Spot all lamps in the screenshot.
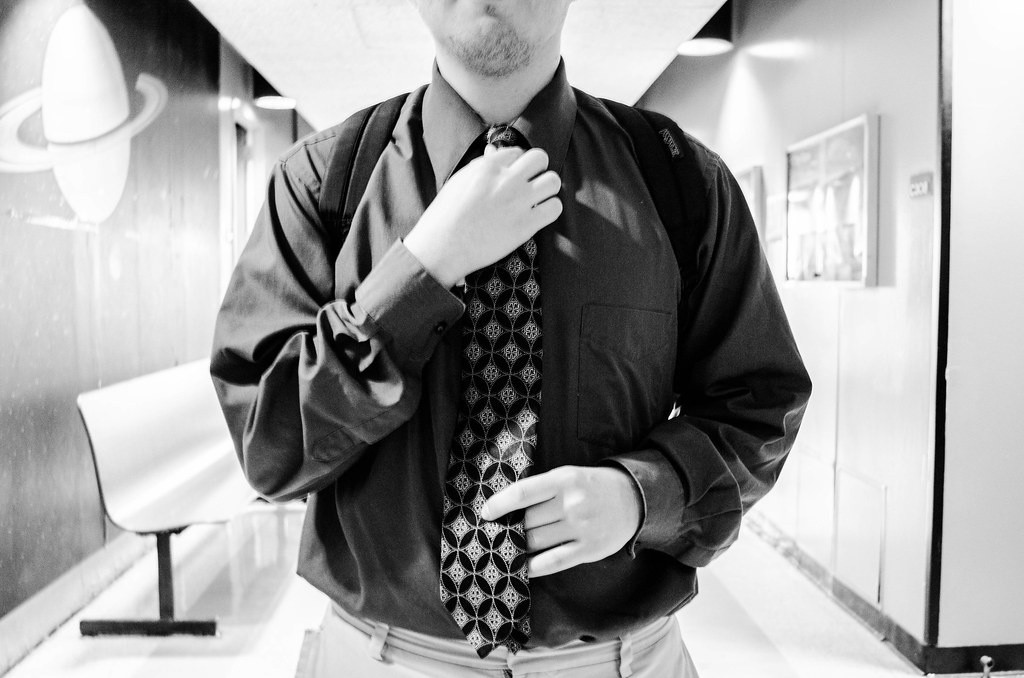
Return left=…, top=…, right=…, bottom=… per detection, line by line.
left=252, top=68, right=296, bottom=110
left=676, top=0, right=733, bottom=57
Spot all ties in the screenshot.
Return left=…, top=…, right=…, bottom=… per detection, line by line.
left=439, top=123, right=545, bottom=659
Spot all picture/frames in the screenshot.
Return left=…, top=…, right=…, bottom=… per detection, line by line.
left=734, top=165, right=762, bottom=237
left=783, top=112, right=878, bottom=291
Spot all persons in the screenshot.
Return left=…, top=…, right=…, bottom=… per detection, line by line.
left=209, top=0, right=812, bottom=677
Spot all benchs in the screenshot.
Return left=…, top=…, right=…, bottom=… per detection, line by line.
left=77, top=357, right=302, bottom=637
